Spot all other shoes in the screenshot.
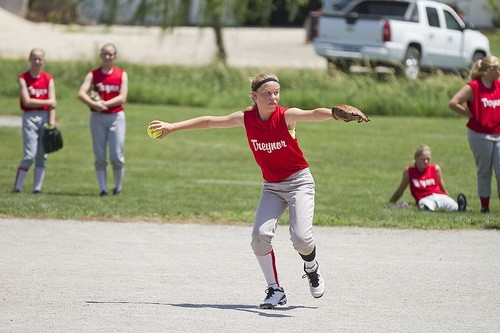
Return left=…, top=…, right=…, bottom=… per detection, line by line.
left=480, top=207, right=491, bottom=212
left=113, top=188, right=123, bottom=195
left=100, top=190, right=108, bottom=196
left=457, top=193, right=467, bottom=212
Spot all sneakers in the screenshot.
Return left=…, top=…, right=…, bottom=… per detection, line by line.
left=302, top=260, right=325, bottom=298
left=260, top=287, right=288, bottom=309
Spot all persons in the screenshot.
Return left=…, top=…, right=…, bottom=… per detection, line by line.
left=78, top=43, right=128, bottom=196
left=449, top=56, right=500, bottom=212
left=12, top=47, right=57, bottom=192
left=389, top=143, right=467, bottom=212
left=148, top=72, right=370, bottom=309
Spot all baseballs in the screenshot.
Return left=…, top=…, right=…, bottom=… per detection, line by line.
left=146, top=123, right=162, bottom=139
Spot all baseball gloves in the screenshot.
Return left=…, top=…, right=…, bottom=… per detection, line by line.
left=41, top=124, right=63, bottom=154
left=332, top=104, right=371, bottom=123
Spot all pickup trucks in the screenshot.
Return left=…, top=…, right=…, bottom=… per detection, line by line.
left=306, top=0, right=490, bottom=82
